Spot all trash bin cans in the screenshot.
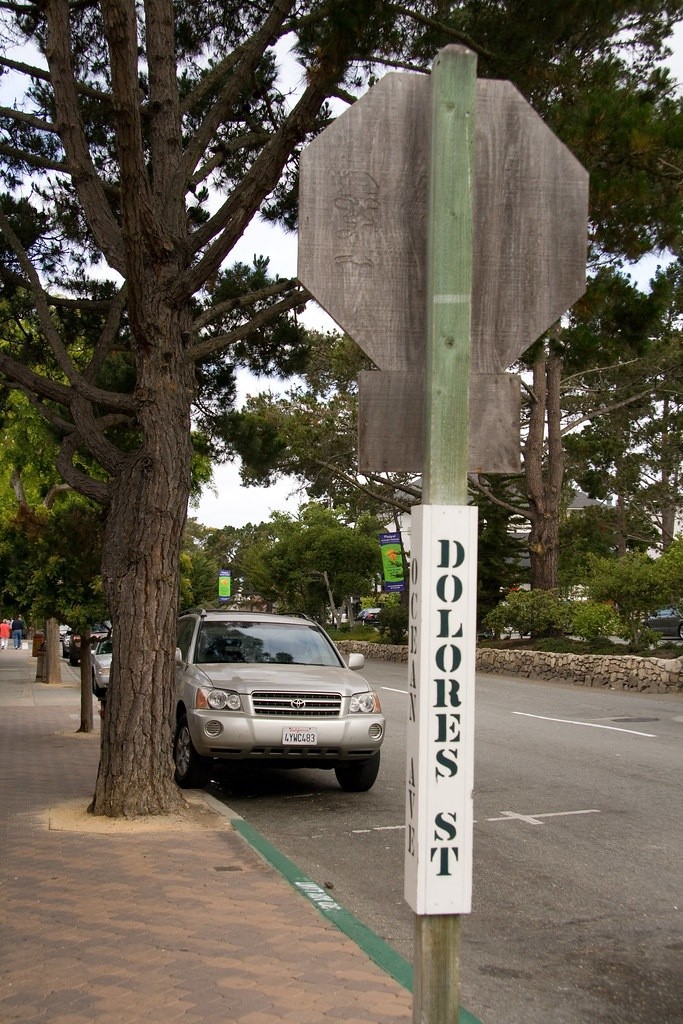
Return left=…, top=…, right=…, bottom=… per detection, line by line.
left=32, top=627, right=44, bottom=657
left=35, top=641, right=47, bottom=682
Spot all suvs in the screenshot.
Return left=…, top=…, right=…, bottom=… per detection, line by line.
left=176, top=608, right=383, bottom=794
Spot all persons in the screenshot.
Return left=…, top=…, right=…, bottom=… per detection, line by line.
left=10, top=616, right=23, bottom=649
left=0, top=619, right=10, bottom=649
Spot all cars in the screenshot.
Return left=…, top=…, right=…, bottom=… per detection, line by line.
left=639, top=608, right=683, bottom=639
left=353, top=608, right=383, bottom=628
left=60, top=624, right=83, bottom=666
left=90, top=635, right=113, bottom=696
